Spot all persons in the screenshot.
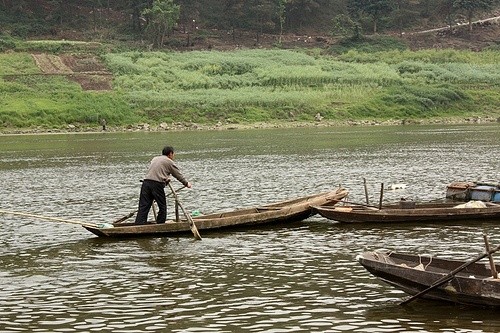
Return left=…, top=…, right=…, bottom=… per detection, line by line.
left=101, top=119, right=107, bottom=130
left=135, top=147, right=191, bottom=224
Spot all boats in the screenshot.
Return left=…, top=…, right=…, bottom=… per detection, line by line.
left=355, top=249, right=500, bottom=309
left=309, top=180, right=500, bottom=222
left=82, top=186, right=349, bottom=236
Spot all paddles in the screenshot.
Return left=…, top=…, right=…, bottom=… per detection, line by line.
left=114, top=186, right=186, bottom=223
left=398, top=245, right=500, bottom=306
left=168, top=182, right=201, bottom=241
left=483, top=234, right=498, bottom=278
